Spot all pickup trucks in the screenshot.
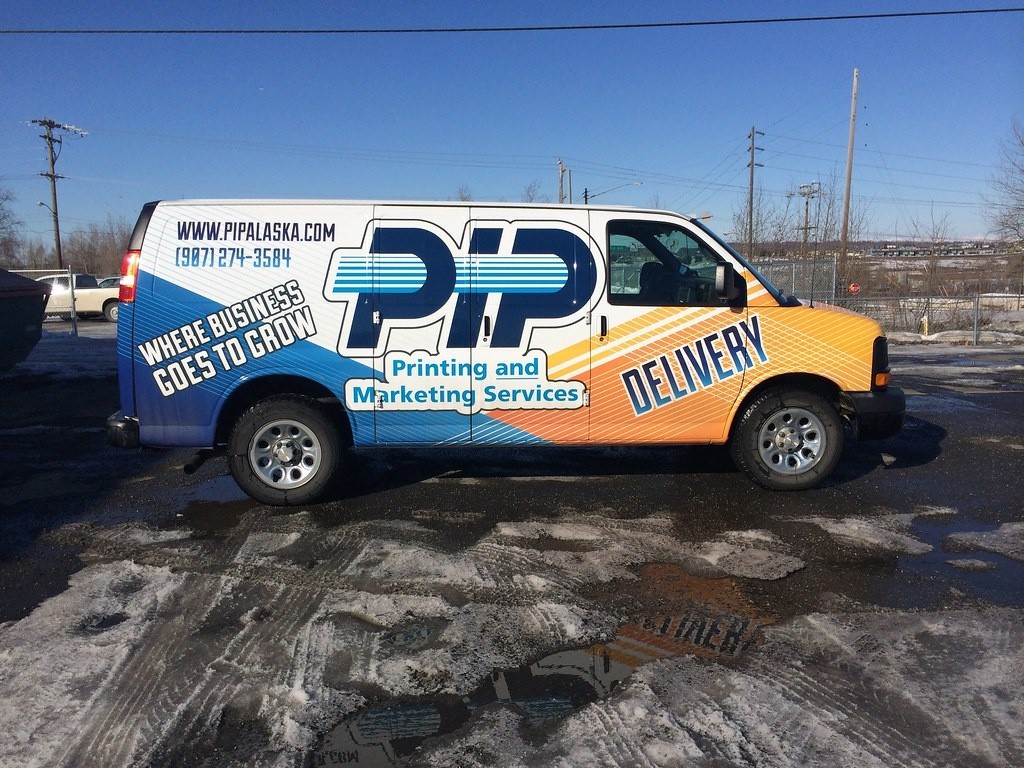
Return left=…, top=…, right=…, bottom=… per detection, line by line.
left=33, top=274, right=120, bottom=323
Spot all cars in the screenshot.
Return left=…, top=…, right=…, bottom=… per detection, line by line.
left=98, top=276, right=120, bottom=288
left=0, top=267, right=53, bottom=371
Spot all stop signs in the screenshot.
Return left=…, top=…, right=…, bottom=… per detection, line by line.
left=849, top=283, right=861, bottom=295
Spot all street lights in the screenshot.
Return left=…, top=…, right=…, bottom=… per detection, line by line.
left=798, top=179, right=821, bottom=261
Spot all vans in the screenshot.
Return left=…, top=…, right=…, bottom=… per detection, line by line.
left=102, top=197, right=892, bottom=506
